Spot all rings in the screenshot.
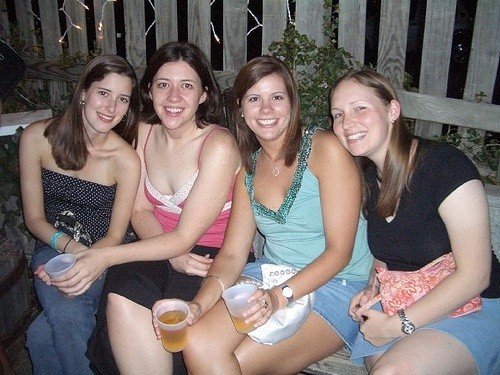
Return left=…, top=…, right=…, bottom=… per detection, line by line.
left=262, top=299, right=268, bottom=309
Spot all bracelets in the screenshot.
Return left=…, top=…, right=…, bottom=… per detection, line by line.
left=62, top=236, right=74, bottom=254
left=50, top=230, right=65, bottom=250
left=201, top=275, right=225, bottom=299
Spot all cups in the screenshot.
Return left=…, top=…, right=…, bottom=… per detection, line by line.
left=44, top=253, right=79, bottom=298
left=222, top=284, right=258, bottom=333
left=154, top=301, right=190, bottom=353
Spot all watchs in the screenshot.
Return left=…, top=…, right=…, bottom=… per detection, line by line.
left=279, top=282, right=295, bottom=306
left=397, top=308, right=415, bottom=335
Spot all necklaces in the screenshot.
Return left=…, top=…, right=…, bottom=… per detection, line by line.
left=261, top=147, right=286, bottom=178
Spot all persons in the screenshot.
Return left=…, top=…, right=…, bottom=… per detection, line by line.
left=104, top=41, right=255, bottom=375
left=326, top=68, right=500, bottom=375
left=151, top=55, right=377, bottom=375
left=16, top=53, right=142, bottom=375
